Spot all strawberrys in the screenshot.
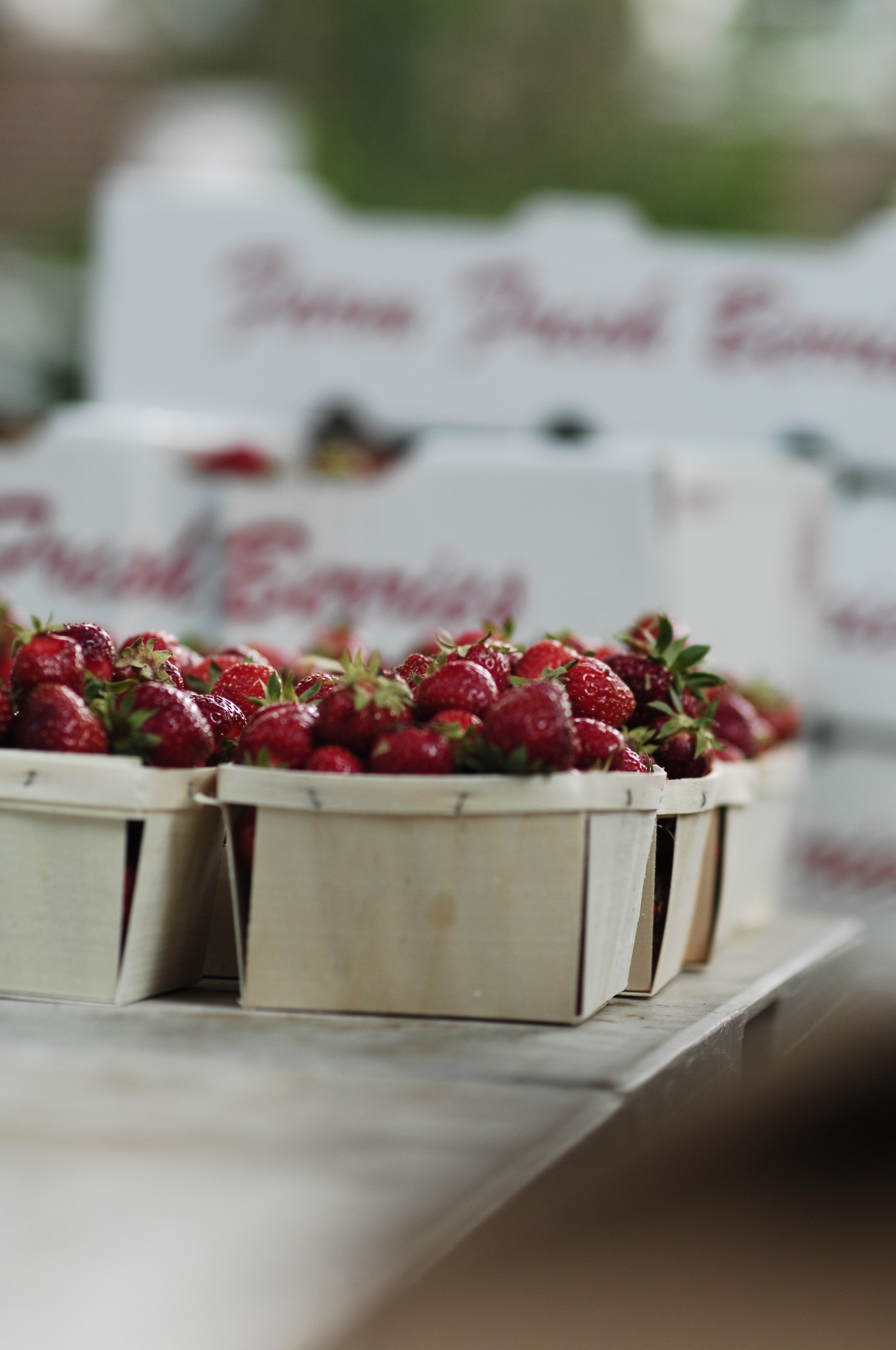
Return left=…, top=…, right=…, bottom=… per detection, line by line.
left=0, top=608, right=802, bottom=774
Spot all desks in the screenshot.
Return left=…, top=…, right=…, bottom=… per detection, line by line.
left=0, top=914, right=877, bottom=1350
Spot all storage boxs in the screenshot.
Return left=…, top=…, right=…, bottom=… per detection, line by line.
left=0, top=735, right=809, bottom=1034
